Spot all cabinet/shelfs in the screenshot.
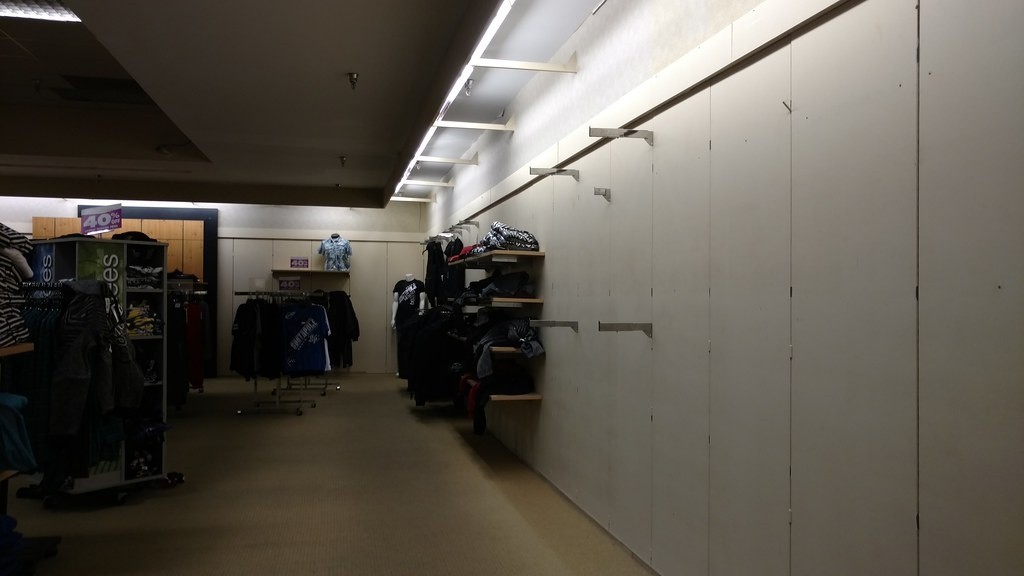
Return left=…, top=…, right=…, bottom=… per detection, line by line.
left=0, top=343, right=36, bottom=516
left=23, top=238, right=170, bottom=494
left=447, top=247, right=546, bottom=401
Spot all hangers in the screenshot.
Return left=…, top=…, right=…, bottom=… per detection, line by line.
left=22, top=281, right=66, bottom=311
left=247, top=291, right=312, bottom=304
